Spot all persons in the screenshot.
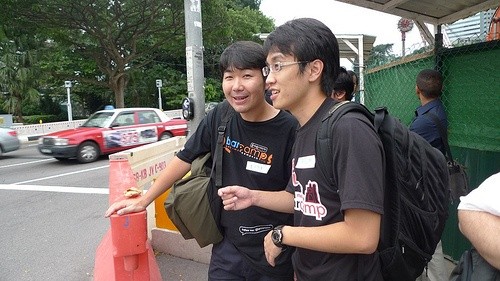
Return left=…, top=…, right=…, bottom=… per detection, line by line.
left=104, top=40, right=299, bottom=281
left=406, top=69, right=448, bottom=159
left=458, top=170, right=500, bottom=271
left=218, top=18, right=389, bottom=281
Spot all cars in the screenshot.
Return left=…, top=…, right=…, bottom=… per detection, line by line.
left=0, top=128, right=22, bottom=159
left=37, top=107, right=187, bottom=164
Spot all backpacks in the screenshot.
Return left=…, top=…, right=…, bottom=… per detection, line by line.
left=318, top=100, right=451, bottom=281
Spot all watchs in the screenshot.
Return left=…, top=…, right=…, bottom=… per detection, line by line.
left=271, top=224, right=285, bottom=247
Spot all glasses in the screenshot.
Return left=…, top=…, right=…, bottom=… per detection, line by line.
left=262, top=61, right=312, bottom=78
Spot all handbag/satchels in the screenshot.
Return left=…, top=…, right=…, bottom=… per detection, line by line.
left=447, top=158, right=469, bottom=205
left=164, top=152, right=223, bottom=249
left=449, top=248, right=500, bottom=281
